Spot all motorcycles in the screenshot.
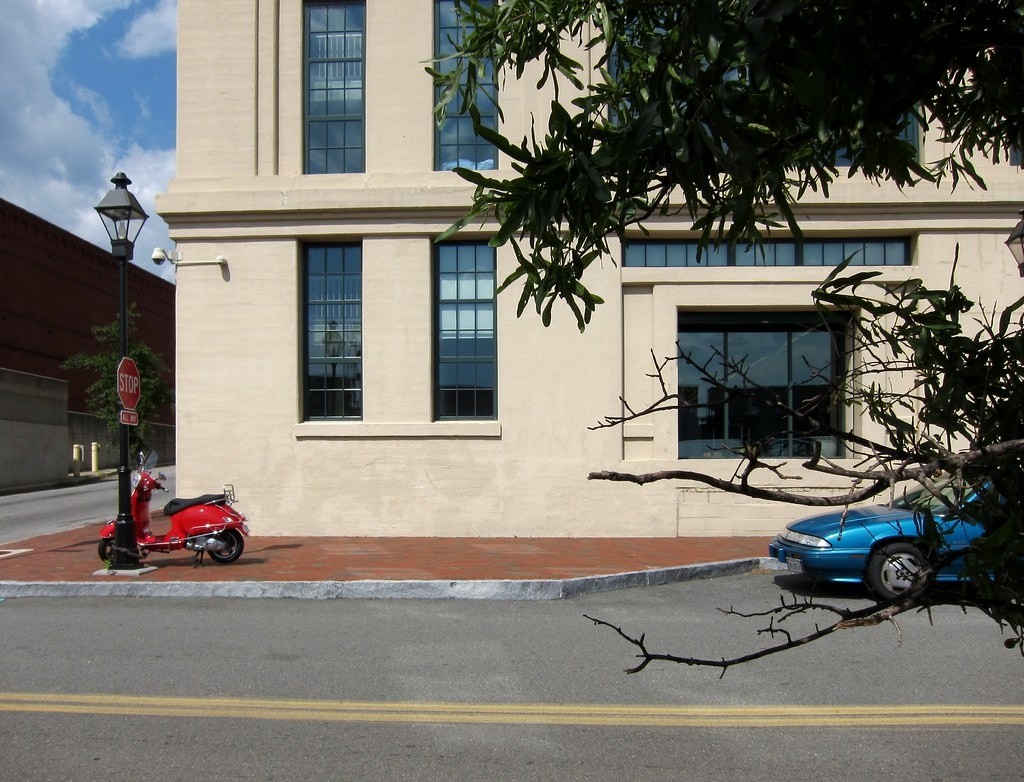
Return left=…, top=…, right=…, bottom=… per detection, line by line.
left=97, top=448, right=251, bottom=571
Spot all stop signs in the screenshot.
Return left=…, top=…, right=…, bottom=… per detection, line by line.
left=117, top=357, right=140, bottom=410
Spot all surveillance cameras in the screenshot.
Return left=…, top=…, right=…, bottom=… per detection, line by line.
left=151, top=251, right=166, bottom=266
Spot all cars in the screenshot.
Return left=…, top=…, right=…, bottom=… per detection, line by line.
left=766, top=471, right=1007, bottom=606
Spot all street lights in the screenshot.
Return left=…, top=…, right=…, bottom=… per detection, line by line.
left=94, top=171, right=152, bottom=572
